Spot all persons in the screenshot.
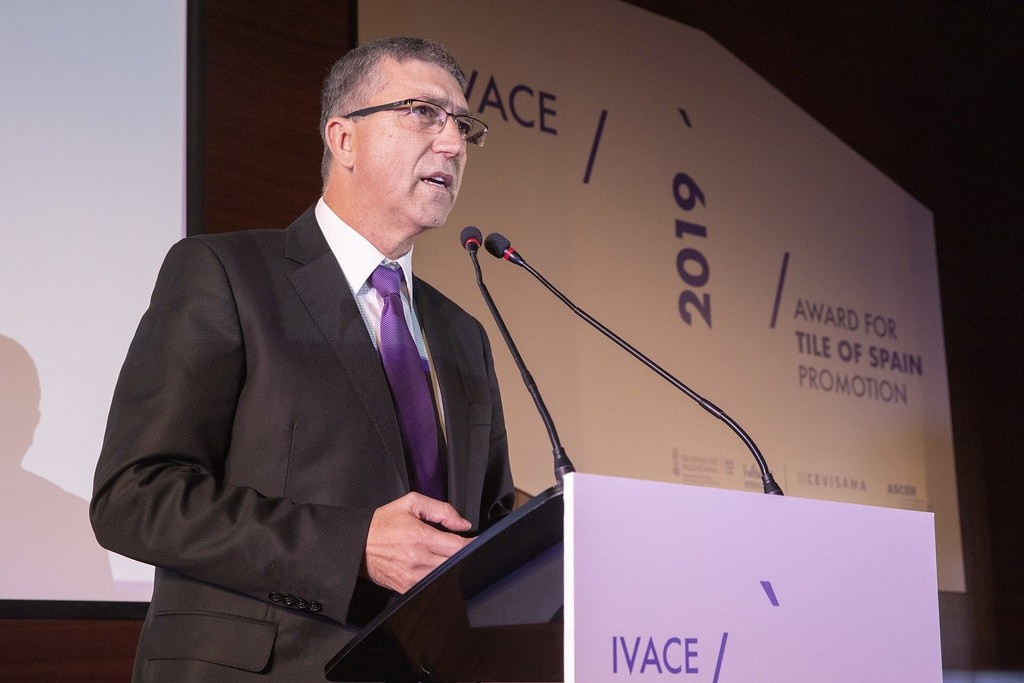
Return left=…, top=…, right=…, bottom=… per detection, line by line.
left=89, top=34, right=517, bottom=683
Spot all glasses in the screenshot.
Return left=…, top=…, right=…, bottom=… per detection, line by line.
left=340, top=99, right=488, bottom=148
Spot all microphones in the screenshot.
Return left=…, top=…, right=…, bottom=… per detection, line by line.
left=459, top=226, right=575, bottom=482
left=484, top=233, right=785, bottom=498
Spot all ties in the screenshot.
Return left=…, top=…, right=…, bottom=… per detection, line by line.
left=368, top=266, right=445, bottom=497
